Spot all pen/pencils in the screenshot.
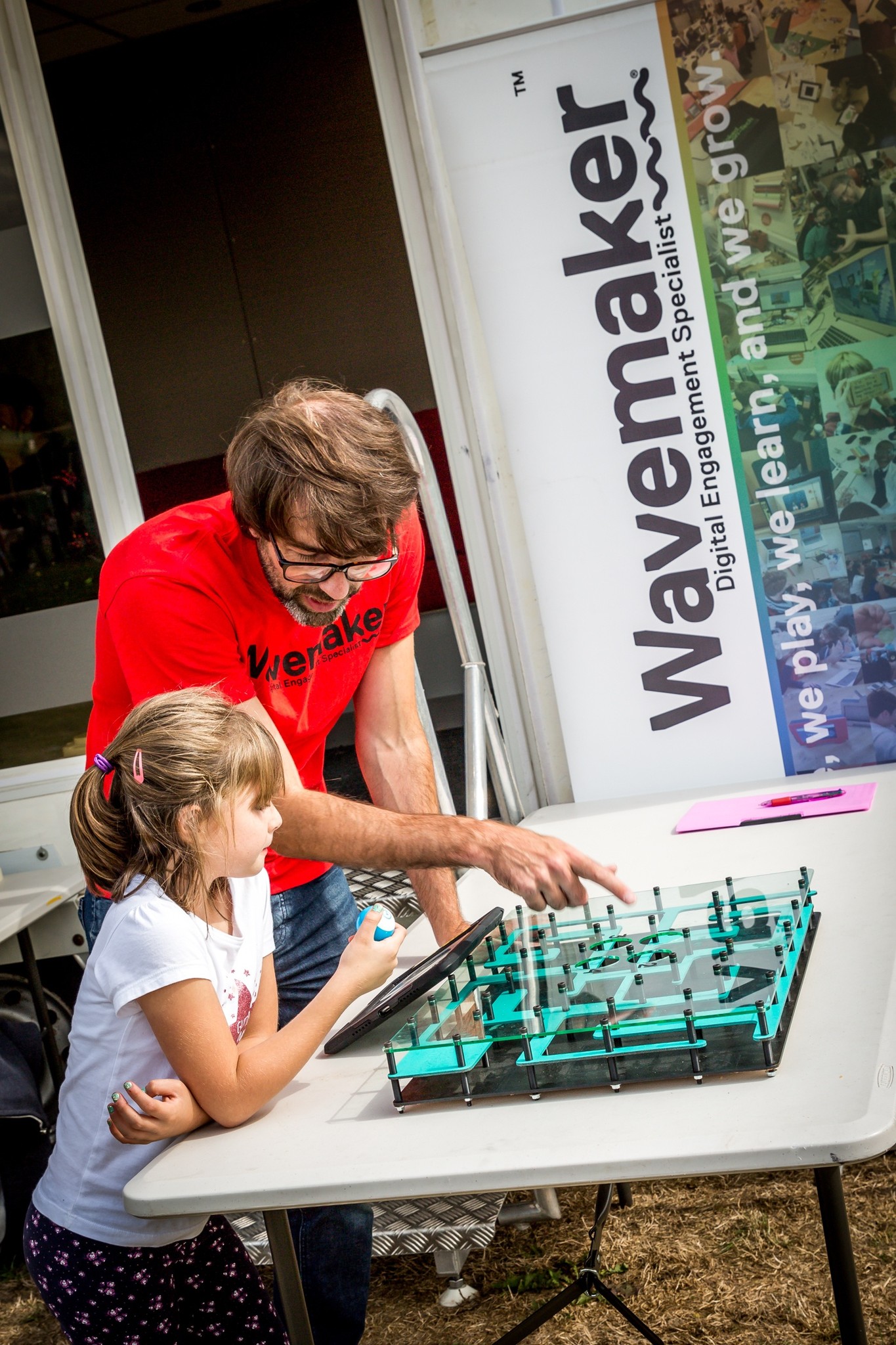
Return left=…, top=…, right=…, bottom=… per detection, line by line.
left=758, top=789, right=846, bottom=807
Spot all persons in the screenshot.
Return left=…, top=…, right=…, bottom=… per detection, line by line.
left=21, top=684, right=409, bottom=1345
left=676, top=0, right=896, bottom=772
left=76, top=377, right=639, bottom=1345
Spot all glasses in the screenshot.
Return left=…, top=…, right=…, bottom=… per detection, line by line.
left=268, top=523, right=398, bottom=582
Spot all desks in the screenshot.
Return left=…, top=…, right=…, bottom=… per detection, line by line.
left=0, top=858, right=88, bottom=1122
left=126, top=766, right=896, bottom=1345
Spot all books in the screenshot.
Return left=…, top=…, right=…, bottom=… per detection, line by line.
left=674, top=782, right=877, bottom=833
